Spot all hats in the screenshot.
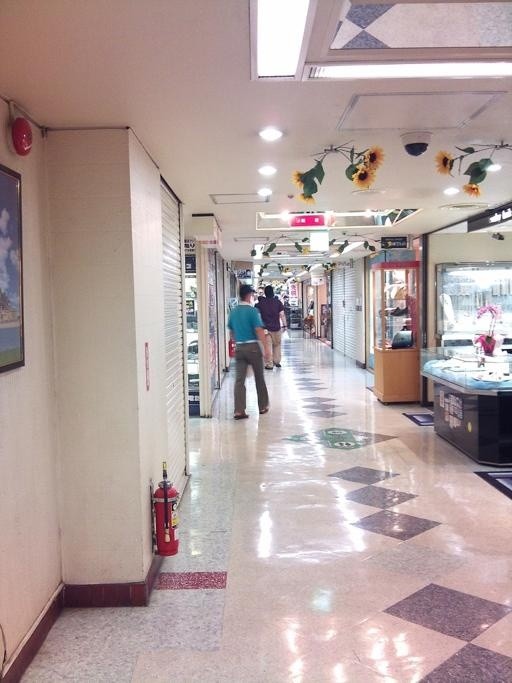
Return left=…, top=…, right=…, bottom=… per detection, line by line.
left=239, top=285, right=257, bottom=295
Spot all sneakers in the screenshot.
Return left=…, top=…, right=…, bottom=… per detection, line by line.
left=275, top=363, right=282, bottom=367
left=264, top=366, right=274, bottom=369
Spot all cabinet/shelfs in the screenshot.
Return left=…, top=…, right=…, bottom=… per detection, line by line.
left=372, top=262, right=420, bottom=403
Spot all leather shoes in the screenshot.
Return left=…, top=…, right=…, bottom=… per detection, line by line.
left=390, top=307, right=409, bottom=316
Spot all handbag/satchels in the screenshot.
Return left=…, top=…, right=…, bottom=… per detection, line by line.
left=392, top=325, right=413, bottom=349
left=390, top=286, right=408, bottom=300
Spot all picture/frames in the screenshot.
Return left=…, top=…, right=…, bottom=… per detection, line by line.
left=0, top=164, right=25, bottom=373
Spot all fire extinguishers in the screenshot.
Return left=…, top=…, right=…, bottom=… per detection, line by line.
left=229, top=337, right=236, bottom=357
left=151, top=462, right=179, bottom=557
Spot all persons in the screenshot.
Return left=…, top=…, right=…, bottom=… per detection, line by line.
left=255, top=286, right=288, bottom=370
left=227, top=284, right=269, bottom=420
left=255, top=295, right=291, bottom=332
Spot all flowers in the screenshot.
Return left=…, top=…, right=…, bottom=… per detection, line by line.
left=290, top=146, right=385, bottom=207
left=252, top=235, right=311, bottom=258
left=327, top=234, right=382, bottom=258
left=434, top=142, right=512, bottom=200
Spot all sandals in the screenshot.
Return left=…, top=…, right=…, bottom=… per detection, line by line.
left=259, top=406, right=270, bottom=414
left=234, top=413, right=249, bottom=420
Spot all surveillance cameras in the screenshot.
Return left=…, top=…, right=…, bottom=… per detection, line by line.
left=491, top=233, right=504, bottom=240
left=400, top=132, right=434, bottom=156
